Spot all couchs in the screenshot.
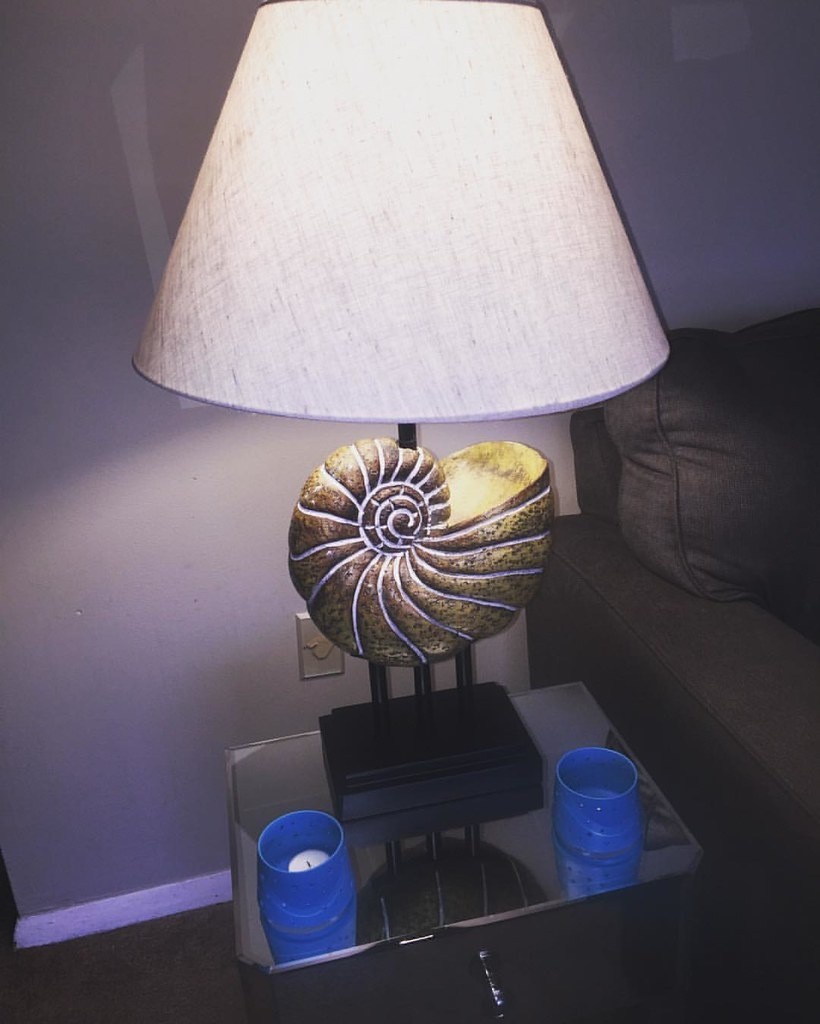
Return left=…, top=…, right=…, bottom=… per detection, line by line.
left=524, top=307, right=820, bottom=1024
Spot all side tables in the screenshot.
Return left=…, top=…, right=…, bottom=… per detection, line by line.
left=225, top=683, right=701, bottom=1024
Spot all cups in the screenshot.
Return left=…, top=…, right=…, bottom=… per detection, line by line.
left=257, top=810, right=357, bottom=930
left=550, top=746, right=643, bottom=857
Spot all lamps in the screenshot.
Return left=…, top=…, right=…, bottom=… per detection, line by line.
left=133, top=0, right=670, bottom=823
